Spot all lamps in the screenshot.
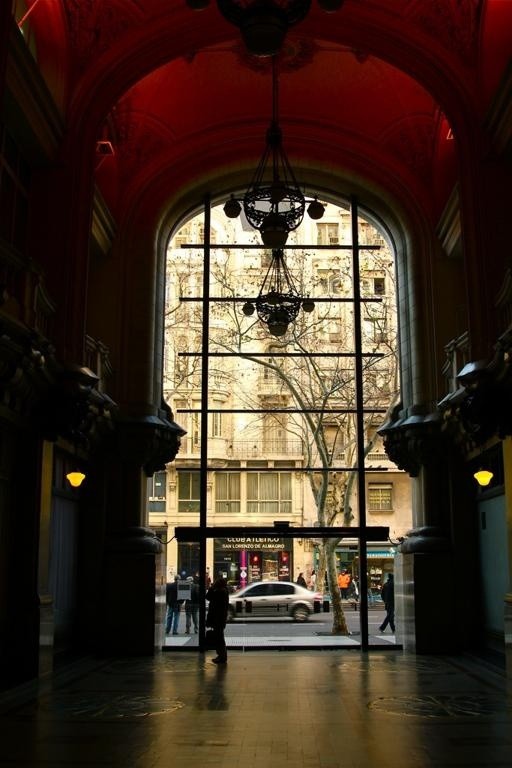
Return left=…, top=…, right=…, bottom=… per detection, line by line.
left=473, top=424, right=495, bottom=487
left=185, top=58, right=345, bottom=246
left=186, top=1, right=345, bottom=58
left=186, top=246, right=345, bottom=335
left=59, top=424, right=89, bottom=488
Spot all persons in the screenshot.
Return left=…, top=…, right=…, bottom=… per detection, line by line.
left=311, top=570, right=317, bottom=585
left=379, top=574, right=395, bottom=633
left=206, top=578, right=229, bottom=664
left=297, top=573, right=307, bottom=589
left=338, top=568, right=351, bottom=599
left=367, top=571, right=373, bottom=603
left=185, top=575, right=200, bottom=634
left=166, top=574, right=184, bottom=635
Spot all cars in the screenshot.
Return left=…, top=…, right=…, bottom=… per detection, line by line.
left=205, top=579, right=326, bottom=625
